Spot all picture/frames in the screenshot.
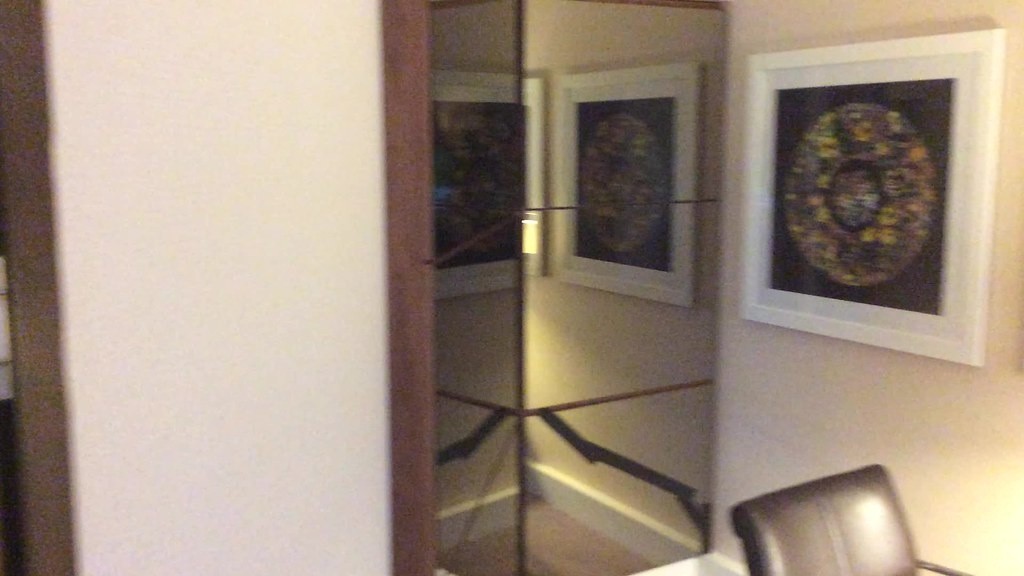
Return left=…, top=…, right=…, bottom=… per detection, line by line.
left=523, top=79, right=549, bottom=286
left=547, top=65, right=705, bottom=304
left=732, top=26, right=1010, bottom=368
left=430, top=71, right=523, bottom=297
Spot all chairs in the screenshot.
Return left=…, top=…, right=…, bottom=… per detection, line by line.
left=728, top=461, right=973, bottom=576
